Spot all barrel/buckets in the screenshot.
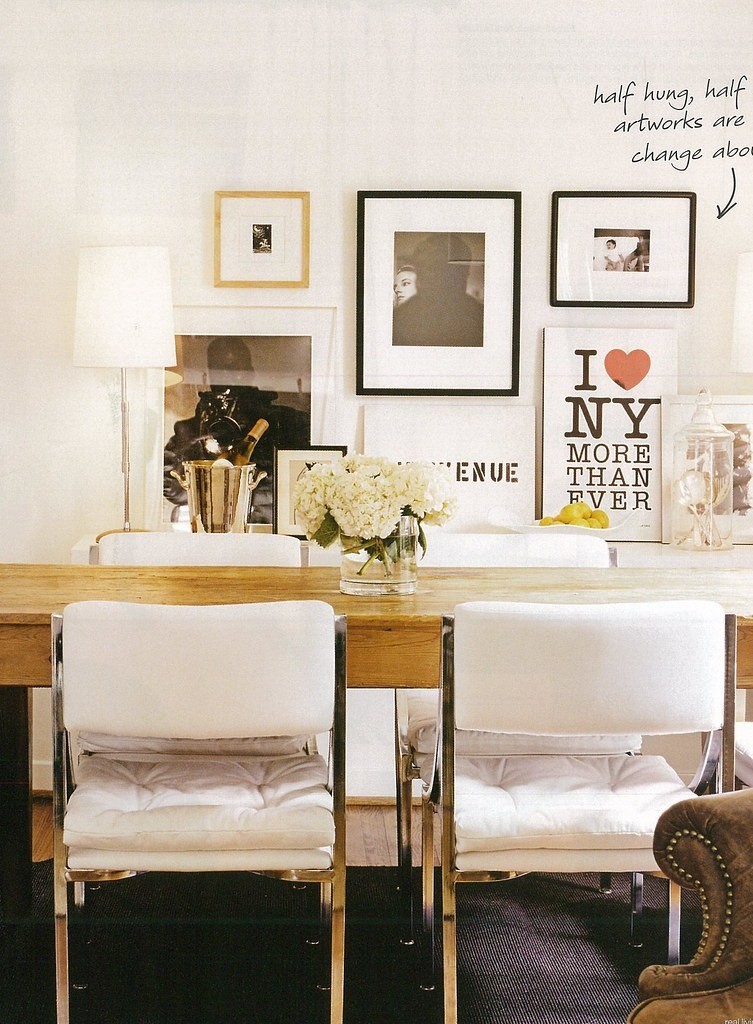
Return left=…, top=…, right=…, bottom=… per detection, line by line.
left=170, top=459, right=267, bottom=534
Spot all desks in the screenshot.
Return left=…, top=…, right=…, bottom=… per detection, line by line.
left=1, top=563, right=753, bottom=957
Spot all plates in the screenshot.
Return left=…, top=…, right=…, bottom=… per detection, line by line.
left=506, top=509, right=636, bottom=540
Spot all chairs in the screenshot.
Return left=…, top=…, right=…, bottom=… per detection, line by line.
left=71, top=531, right=321, bottom=945
left=421, top=601, right=735, bottom=1024
left=52, top=599, right=347, bottom=1024
left=392, top=530, right=650, bottom=950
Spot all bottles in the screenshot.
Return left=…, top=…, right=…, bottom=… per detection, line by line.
left=210, top=419, right=269, bottom=467
left=671, top=386, right=734, bottom=551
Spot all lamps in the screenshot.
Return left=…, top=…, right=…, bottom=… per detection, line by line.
left=71, top=245, right=179, bottom=542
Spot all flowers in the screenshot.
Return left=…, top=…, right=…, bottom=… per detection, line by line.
left=293, top=454, right=460, bottom=581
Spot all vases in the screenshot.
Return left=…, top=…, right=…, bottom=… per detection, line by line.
left=339, top=516, right=419, bottom=595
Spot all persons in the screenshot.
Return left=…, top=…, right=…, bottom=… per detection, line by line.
left=165, top=337, right=310, bottom=527
left=604, top=240, right=624, bottom=271
left=392, top=233, right=484, bottom=348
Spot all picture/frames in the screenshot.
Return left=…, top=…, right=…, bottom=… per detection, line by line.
left=549, top=191, right=696, bottom=308
left=214, top=190, right=310, bottom=287
left=358, top=190, right=522, bottom=398
